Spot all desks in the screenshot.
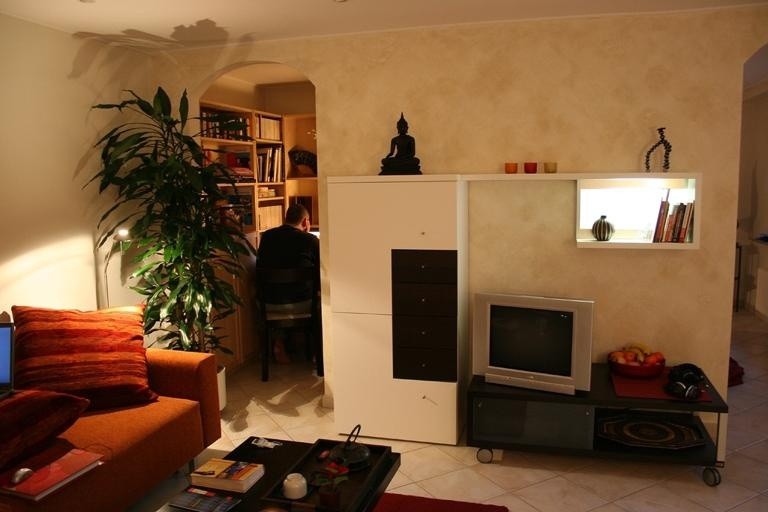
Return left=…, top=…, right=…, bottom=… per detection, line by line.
left=462, top=363, right=731, bottom=490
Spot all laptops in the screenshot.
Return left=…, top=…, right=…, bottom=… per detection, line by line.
left=0, top=322, right=15, bottom=399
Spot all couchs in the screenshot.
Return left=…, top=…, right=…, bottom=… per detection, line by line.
left=0, top=343, right=222, bottom=510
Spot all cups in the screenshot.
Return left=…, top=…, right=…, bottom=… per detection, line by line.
left=281, top=473, right=307, bottom=499
left=505, top=162, right=557, bottom=174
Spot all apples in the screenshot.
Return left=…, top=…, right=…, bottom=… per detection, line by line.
left=645, top=356, right=656, bottom=366
left=651, top=352, right=664, bottom=362
left=609, top=351, right=640, bottom=366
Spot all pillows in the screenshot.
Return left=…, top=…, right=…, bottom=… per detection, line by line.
left=0, top=302, right=159, bottom=473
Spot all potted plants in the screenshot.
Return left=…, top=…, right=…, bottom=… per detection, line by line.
left=78, top=84, right=259, bottom=422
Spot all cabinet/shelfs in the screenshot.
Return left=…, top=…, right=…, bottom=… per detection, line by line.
left=197, top=97, right=319, bottom=377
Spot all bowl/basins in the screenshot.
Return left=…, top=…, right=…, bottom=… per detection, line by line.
left=608, top=350, right=666, bottom=381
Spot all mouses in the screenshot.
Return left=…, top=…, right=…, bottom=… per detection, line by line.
left=11, top=468, right=33, bottom=484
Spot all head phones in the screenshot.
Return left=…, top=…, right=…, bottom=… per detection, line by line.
left=667, top=363, right=705, bottom=400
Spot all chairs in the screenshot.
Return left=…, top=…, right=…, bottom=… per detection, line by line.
left=252, top=264, right=322, bottom=382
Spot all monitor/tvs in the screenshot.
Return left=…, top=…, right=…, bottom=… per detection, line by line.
left=471, top=292, right=595, bottom=396
left=308, top=224, right=320, bottom=239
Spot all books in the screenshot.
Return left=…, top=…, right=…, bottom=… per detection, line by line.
left=0, top=445, right=106, bottom=502
left=166, top=457, right=265, bottom=512
left=651, top=200, right=694, bottom=243
left=201, top=111, right=313, bottom=229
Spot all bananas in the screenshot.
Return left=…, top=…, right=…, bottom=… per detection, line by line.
left=623, top=343, right=651, bottom=363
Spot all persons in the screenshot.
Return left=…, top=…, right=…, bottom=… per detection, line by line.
left=257, top=203, right=321, bottom=364
left=378, top=112, right=429, bottom=173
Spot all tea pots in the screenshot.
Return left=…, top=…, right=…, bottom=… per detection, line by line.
left=327, top=425, right=373, bottom=476
